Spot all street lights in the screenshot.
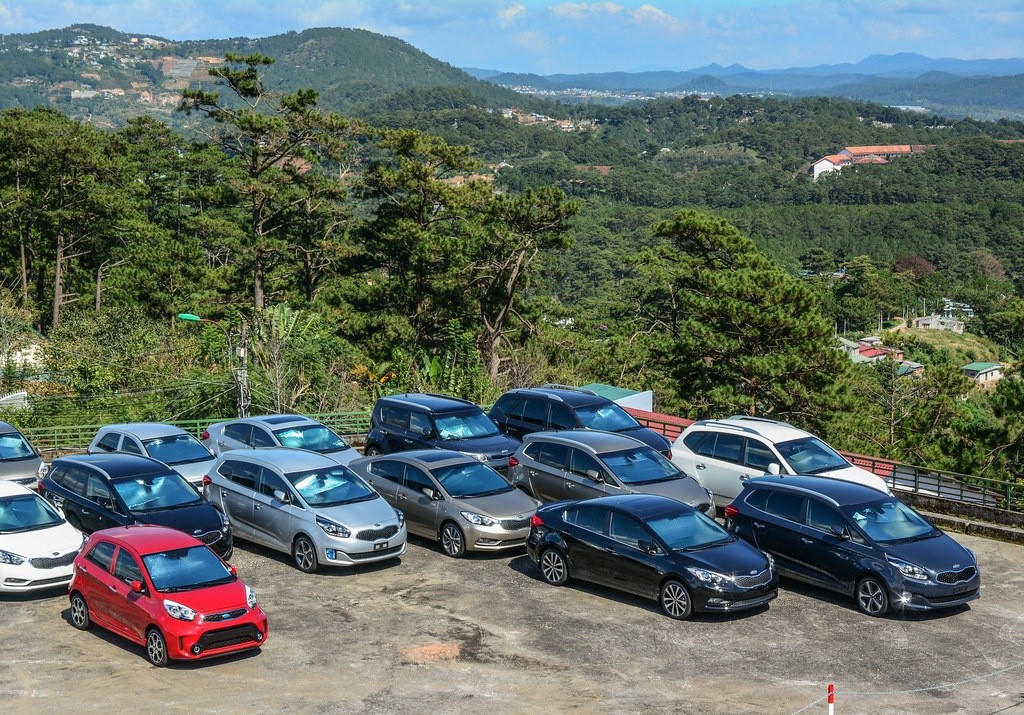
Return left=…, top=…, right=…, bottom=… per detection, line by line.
left=178, top=312, right=249, bottom=418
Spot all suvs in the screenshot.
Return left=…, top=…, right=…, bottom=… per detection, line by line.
left=515, top=427, right=718, bottom=520
left=362, top=393, right=524, bottom=479
left=37, top=450, right=232, bottom=572
left=484, top=384, right=673, bottom=466
left=671, top=416, right=894, bottom=514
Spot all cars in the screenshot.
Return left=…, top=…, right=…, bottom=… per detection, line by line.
left=0, top=479, right=86, bottom=594
left=84, top=422, right=219, bottom=493
left=68, top=523, right=269, bottom=667
left=199, top=446, right=408, bottom=572
left=0, top=421, right=50, bottom=491
left=523, top=493, right=781, bottom=621
left=343, top=450, right=543, bottom=558
left=199, top=413, right=371, bottom=487
left=723, top=475, right=980, bottom=616
